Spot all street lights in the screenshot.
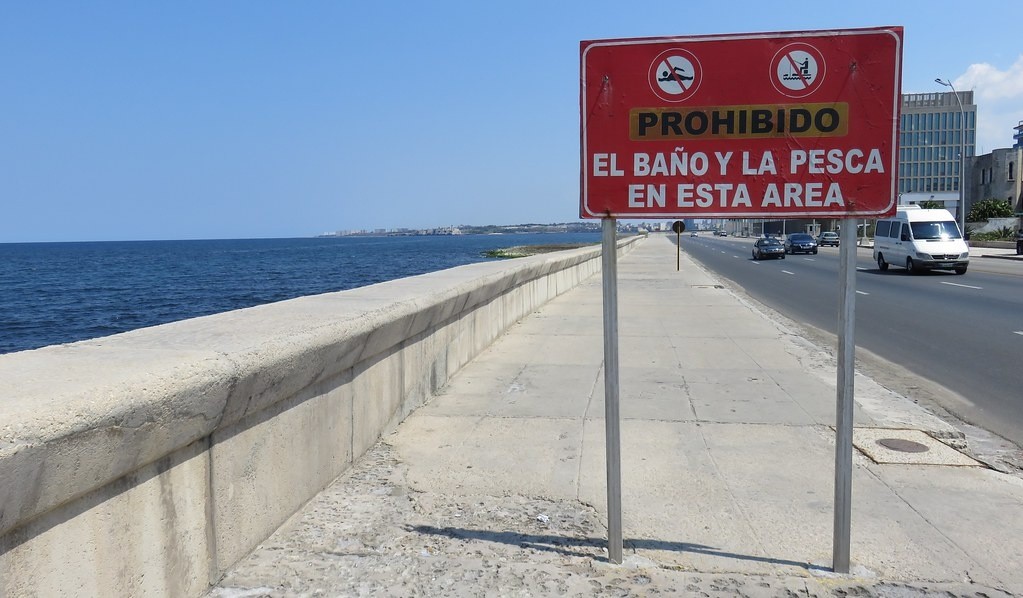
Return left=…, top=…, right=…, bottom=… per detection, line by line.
left=935, top=77, right=965, bottom=239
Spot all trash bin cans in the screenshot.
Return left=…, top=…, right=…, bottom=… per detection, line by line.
left=1017, top=240, right=1023, bottom=255
left=965, top=232, right=970, bottom=240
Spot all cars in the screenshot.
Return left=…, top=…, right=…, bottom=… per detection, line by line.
left=719, top=231, right=727, bottom=237
left=784, top=233, right=819, bottom=255
left=751, top=238, right=786, bottom=260
left=691, top=231, right=698, bottom=237
left=816, top=232, right=840, bottom=247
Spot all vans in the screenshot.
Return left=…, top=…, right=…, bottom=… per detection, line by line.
left=874, top=204, right=970, bottom=274
